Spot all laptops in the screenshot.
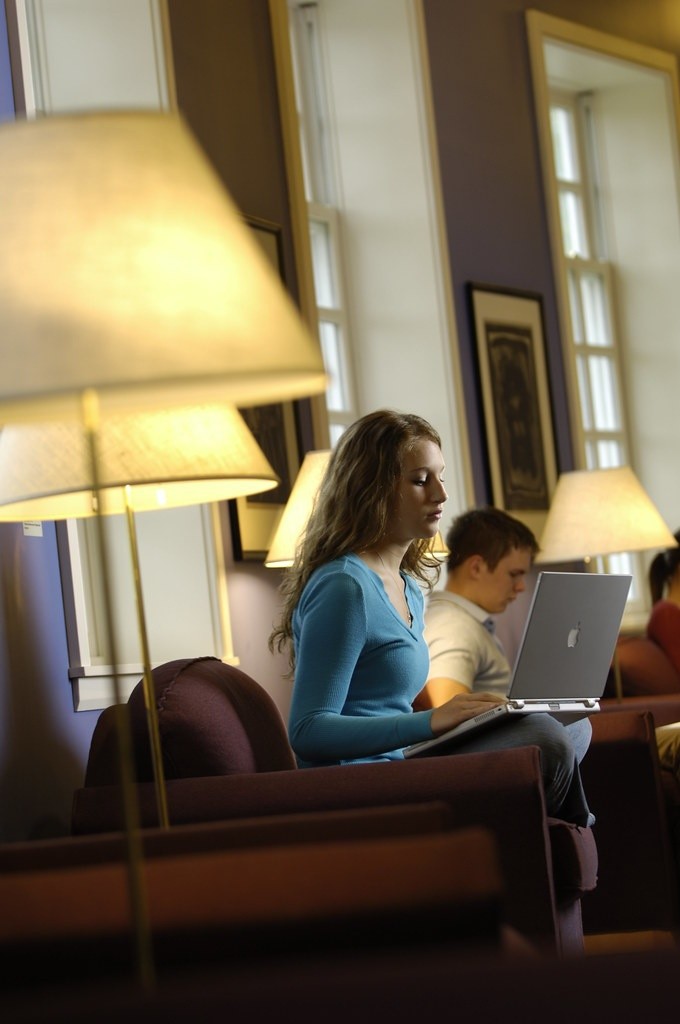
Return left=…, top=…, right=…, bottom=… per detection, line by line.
left=401, top=571, right=633, bottom=760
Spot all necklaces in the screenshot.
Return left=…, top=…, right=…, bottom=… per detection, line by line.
left=374, top=549, right=412, bottom=623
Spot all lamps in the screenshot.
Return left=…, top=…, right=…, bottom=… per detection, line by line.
left=0, top=113, right=329, bottom=1024
left=532, top=466, right=677, bottom=708
left=0, top=404, right=279, bottom=826
left=265, top=449, right=450, bottom=568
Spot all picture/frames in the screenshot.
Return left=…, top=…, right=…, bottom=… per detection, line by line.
left=227, top=214, right=316, bottom=564
left=465, top=282, right=559, bottom=565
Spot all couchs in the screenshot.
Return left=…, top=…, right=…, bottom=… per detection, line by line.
left=0, top=523, right=680, bottom=1024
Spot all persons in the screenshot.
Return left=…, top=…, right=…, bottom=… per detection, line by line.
left=284, top=411, right=598, bottom=829
left=412, top=505, right=679, bottom=779
